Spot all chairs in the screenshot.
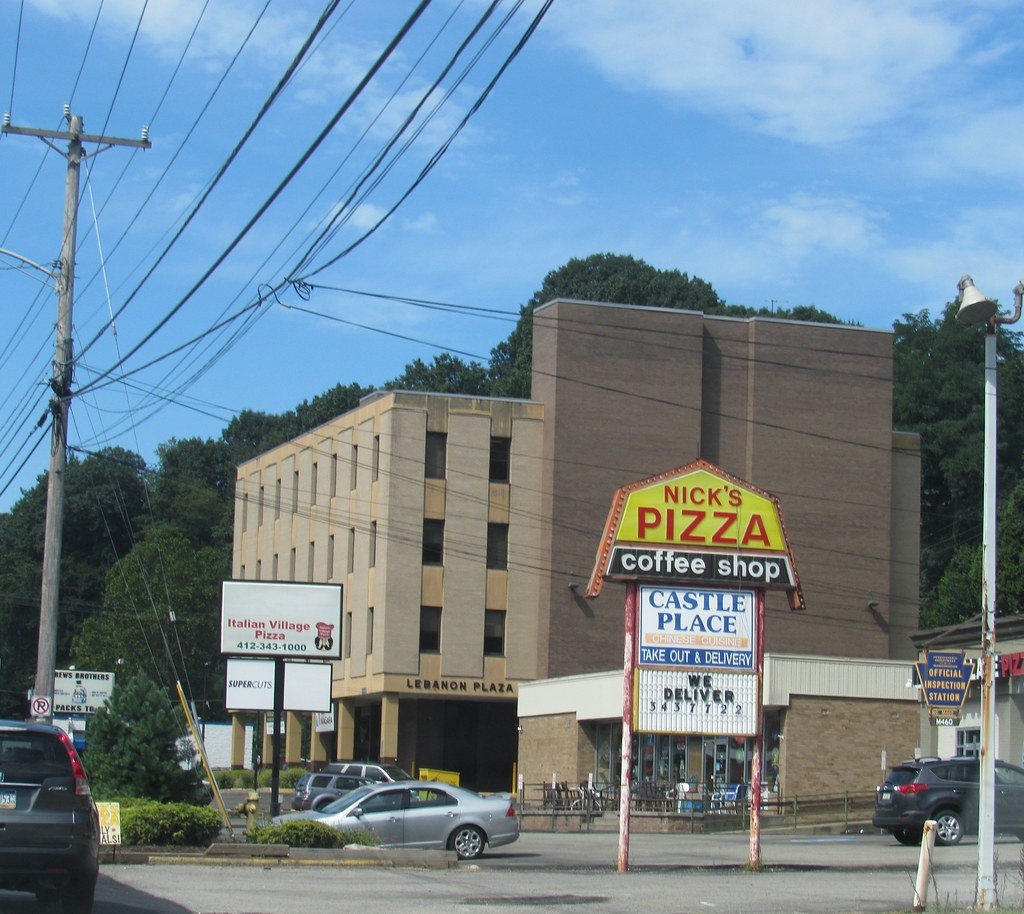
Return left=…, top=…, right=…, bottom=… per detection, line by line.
left=548, top=771, right=741, bottom=814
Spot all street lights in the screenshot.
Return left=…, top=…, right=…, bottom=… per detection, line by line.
left=953, top=274, right=1024, bottom=909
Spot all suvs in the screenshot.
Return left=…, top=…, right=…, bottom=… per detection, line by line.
left=321, top=758, right=417, bottom=794
left=870, top=755, right=1024, bottom=846
left=290, top=771, right=420, bottom=814
left=0, top=718, right=102, bottom=914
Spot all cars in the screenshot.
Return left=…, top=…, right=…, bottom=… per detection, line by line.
left=258, top=779, right=520, bottom=861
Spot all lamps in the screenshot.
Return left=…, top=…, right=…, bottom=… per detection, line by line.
left=567, top=570, right=579, bottom=589
left=867, top=589, right=878, bottom=607
